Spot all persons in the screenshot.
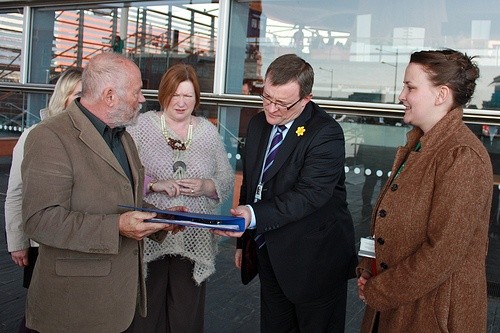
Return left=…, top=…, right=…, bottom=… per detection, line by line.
left=123, top=63, right=234, bottom=333
left=355, top=49, right=493, bottom=333
left=239, top=79, right=260, bottom=137
left=209, top=53, right=360, bottom=333
left=3, top=64, right=84, bottom=333
left=357, top=102, right=481, bottom=219
left=20, top=53, right=190, bottom=333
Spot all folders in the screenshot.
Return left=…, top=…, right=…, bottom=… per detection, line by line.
left=117, top=204, right=245, bottom=232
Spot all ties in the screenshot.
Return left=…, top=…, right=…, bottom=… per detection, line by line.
left=254, top=124, right=286, bottom=249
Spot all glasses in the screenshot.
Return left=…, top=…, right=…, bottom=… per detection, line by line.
left=260, top=85, right=304, bottom=109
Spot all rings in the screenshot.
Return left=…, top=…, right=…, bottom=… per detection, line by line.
left=192, top=189, right=194, bottom=192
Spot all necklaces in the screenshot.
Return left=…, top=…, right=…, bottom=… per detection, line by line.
left=161, top=110, right=193, bottom=180
